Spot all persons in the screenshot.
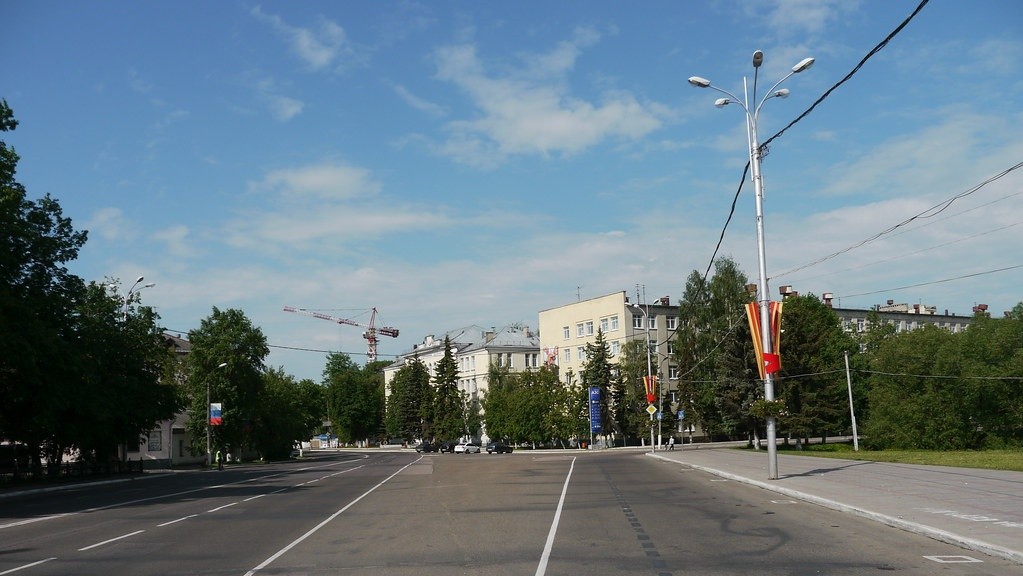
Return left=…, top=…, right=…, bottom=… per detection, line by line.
left=216, top=448, right=241, bottom=471
left=665, top=436, right=675, bottom=451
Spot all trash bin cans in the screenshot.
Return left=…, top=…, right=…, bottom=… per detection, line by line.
left=582, top=442, right=587, bottom=449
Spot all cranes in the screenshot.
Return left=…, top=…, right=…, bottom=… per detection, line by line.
left=282, top=303, right=400, bottom=363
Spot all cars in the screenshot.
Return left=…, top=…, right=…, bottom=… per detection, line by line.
left=440, top=441, right=459, bottom=453
left=454, top=442, right=480, bottom=454
left=486, top=441, right=514, bottom=454
left=416, top=441, right=439, bottom=454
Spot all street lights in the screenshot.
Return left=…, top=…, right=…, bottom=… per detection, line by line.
left=686, top=49, right=816, bottom=481
left=658, top=357, right=670, bottom=450
left=623, top=298, right=661, bottom=452
left=204, top=362, right=228, bottom=466
left=121, top=274, right=156, bottom=475
